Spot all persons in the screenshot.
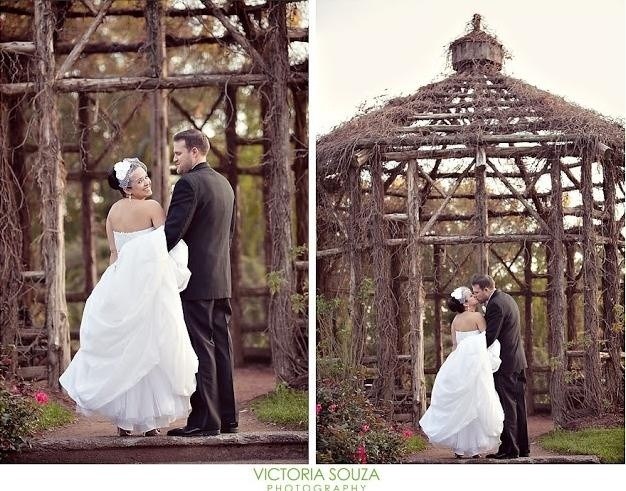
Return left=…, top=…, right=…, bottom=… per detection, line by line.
left=418, top=287, right=506, bottom=458
left=163, top=129, right=240, bottom=437
left=59, top=159, right=200, bottom=436
left=470, top=272, right=530, bottom=459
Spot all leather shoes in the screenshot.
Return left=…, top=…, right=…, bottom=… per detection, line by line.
left=486, top=451, right=530, bottom=458
left=167, top=421, right=238, bottom=437
